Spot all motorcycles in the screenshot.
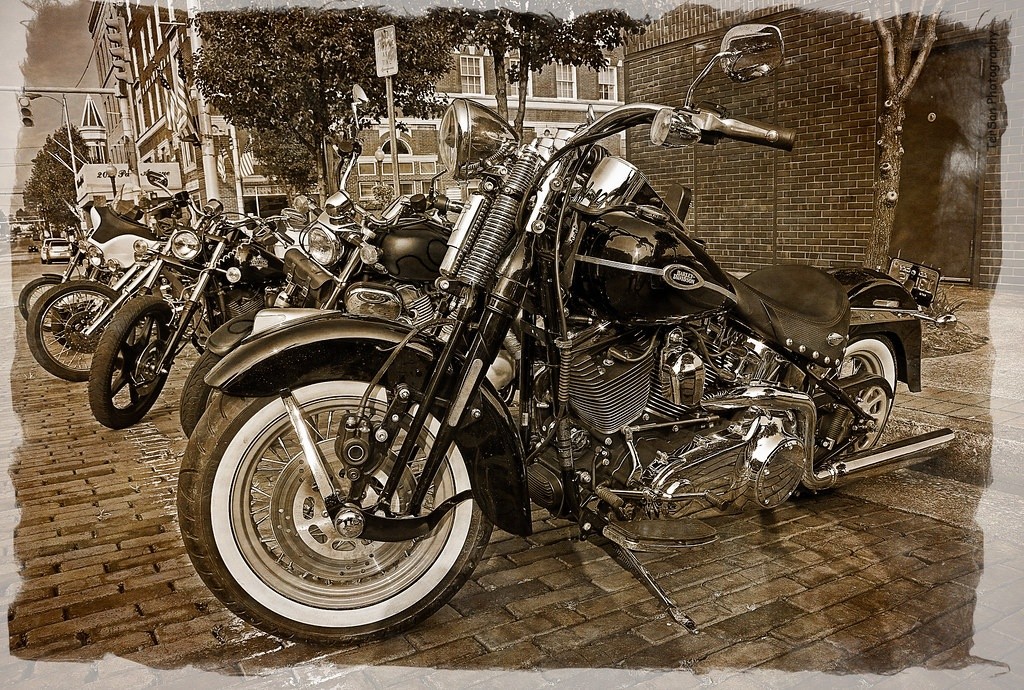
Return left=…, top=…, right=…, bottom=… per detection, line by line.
left=17, top=24, right=959, bottom=650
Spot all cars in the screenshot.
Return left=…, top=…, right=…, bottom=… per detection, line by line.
left=47, top=240, right=71, bottom=264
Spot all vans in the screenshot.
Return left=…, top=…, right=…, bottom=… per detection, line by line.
left=40, top=238, right=67, bottom=264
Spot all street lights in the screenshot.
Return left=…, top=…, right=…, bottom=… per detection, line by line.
left=373, top=146, right=385, bottom=210
left=25, top=92, right=87, bottom=237
left=105, top=163, right=117, bottom=199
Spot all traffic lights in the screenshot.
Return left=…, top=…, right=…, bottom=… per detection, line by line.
left=17, top=96, right=34, bottom=128
left=104, top=17, right=132, bottom=62
left=112, top=60, right=134, bottom=83
